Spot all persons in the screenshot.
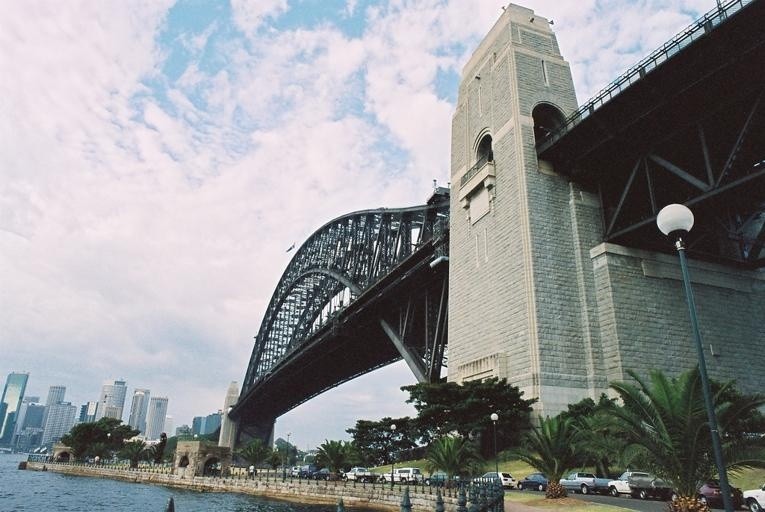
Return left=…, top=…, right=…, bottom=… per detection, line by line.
left=249, top=464, right=254, bottom=480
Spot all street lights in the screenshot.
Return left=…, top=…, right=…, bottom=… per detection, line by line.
left=491, top=413, right=499, bottom=477
left=656, top=202, right=734, bottom=510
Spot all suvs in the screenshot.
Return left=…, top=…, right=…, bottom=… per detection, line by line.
left=379, top=468, right=423, bottom=485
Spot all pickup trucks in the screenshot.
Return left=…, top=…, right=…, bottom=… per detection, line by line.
left=342, top=469, right=380, bottom=484
left=559, top=472, right=614, bottom=495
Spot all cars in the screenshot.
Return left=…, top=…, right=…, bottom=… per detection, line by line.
left=741, top=484, right=765, bottom=512
left=517, top=473, right=551, bottom=492
left=425, top=473, right=460, bottom=486
left=288, top=467, right=330, bottom=480
left=608, top=472, right=673, bottom=500
left=671, top=479, right=743, bottom=509
left=474, top=472, right=516, bottom=489
